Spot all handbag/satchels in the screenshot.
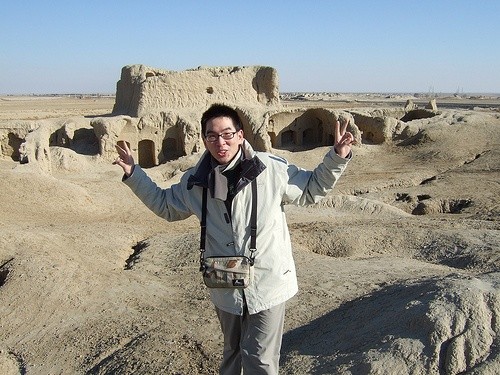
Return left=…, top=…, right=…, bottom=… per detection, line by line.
left=203, top=256, right=254, bottom=289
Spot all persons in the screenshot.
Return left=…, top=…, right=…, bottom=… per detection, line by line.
left=112, top=105, right=354, bottom=375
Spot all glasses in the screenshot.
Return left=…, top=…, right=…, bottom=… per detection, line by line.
left=202, top=131, right=238, bottom=142
